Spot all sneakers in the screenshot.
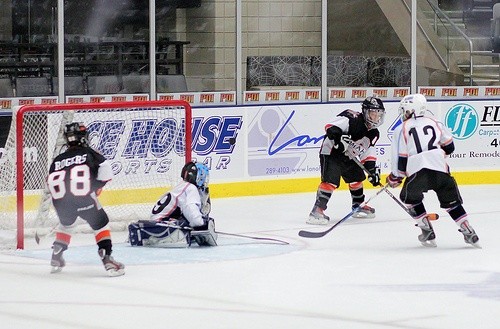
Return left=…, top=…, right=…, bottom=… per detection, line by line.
left=98, top=249, right=125, bottom=276
left=128, top=223, right=143, bottom=247
left=49, top=244, right=66, bottom=273
left=306, top=206, right=330, bottom=224
left=352, top=201, right=375, bottom=217
left=458, top=226, right=479, bottom=246
left=418, top=222, right=437, bottom=246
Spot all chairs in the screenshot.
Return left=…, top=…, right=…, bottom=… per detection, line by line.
left=0, top=74, right=188, bottom=97
left=438, top=0, right=500, bottom=63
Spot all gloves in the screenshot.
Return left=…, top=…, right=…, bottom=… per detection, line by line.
left=363, top=161, right=381, bottom=186
left=332, top=135, right=352, bottom=153
left=386, top=172, right=404, bottom=188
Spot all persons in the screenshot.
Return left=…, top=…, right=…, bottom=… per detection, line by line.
left=47, top=123, right=125, bottom=277
left=386, top=95, right=482, bottom=248
left=150, top=163, right=217, bottom=246
left=306, top=96, right=384, bottom=225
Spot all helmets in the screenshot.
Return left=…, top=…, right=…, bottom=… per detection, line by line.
left=361, top=97, right=385, bottom=129
left=399, top=94, right=426, bottom=121
left=63, top=122, right=90, bottom=143
left=181, top=162, right=209, bottom=187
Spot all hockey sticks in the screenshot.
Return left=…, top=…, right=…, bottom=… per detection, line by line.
left=152, top=222, right=289, bottom=245
left=298, top=183, right=390, bottom=238
left=346, top=149, right=440, bottom=221
left=34, top=177, right=113, bottom=246
